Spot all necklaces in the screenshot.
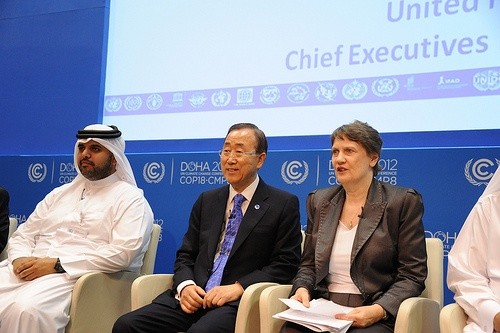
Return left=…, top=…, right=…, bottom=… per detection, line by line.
left=349, top=222, right=352, bottom=228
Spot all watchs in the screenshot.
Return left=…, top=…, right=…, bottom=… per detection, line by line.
left=54, top=258, right=66, bottom=273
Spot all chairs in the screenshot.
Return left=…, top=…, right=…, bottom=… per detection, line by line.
left=130, top=230, right=305, bottom=333
left=259, top=238, right=444, bottom=333
left=64, top=223, right=162, bottom=333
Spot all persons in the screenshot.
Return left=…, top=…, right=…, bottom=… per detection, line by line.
left=280, top=120, right=428, bottom=333
left=112, top=124, right=303, bottom=333
left=446, top=165, right=500, bottom=333
left=0, top=124, right=154, bottom=333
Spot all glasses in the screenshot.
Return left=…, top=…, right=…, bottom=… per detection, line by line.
left=219, top=150, right=262, bottom=158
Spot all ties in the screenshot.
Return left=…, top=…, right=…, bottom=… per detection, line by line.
left=206, top=193, right=247, bottom=292
